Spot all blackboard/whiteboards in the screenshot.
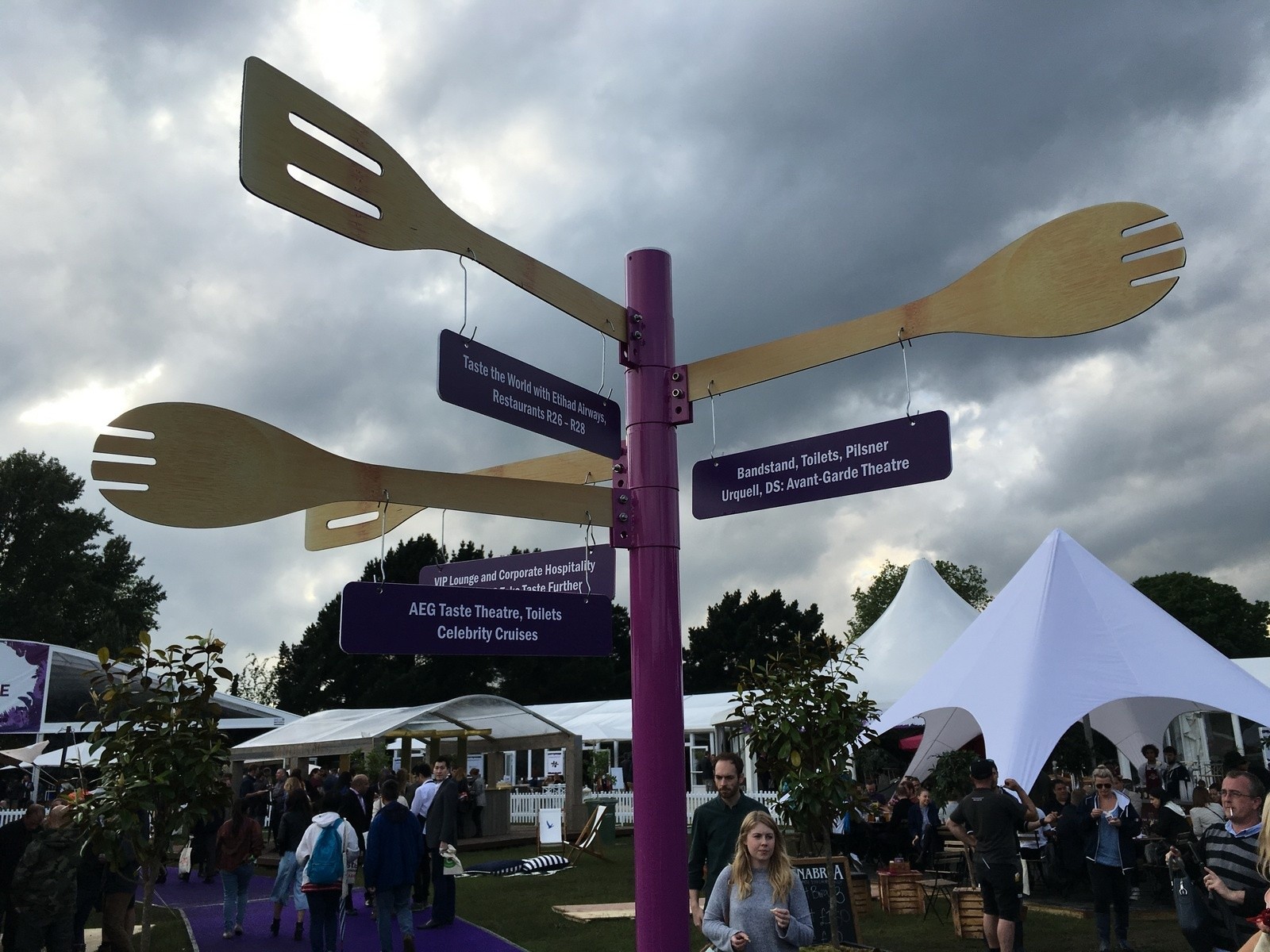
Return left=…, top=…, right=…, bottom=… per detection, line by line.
left=789, top=856, right=863, bottom=945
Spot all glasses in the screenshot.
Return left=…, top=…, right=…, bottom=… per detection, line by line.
left=1096, top=783, right=1111, bottom=788
left=1221, top=790, right=1253, bottom=798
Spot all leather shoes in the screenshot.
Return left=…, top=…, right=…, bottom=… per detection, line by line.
left=417, top=919, right=445, bottom=929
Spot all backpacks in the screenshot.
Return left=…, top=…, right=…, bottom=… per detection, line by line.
left=307, top=819, right=343, bottom=885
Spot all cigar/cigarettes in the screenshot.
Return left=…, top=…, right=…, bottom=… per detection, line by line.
left=1230, top=807, right=1233, bottom=817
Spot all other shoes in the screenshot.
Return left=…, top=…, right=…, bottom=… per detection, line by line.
left=362, top=891, right=376, bottom=907
left=407, top=903, right=430, bottom=912
left=1129, top=887, right=1142, bottom=900
left=346, top=908, right=358, bottom=915
left=851, top=863, right=860, bottom=872
left=370, top=913, right=376, bottom=920
left=850, top=852, right=862, bottom=866
left=471, top=835, right=483, bottom=839
left=404, top=936, right=414, bottom=952
left=222, top=925, right=242, bottom=939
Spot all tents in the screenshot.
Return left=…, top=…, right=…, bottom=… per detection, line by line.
left=715, top=526, right=1270, bottom=832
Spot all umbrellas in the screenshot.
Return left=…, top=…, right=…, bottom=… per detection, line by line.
left=0, top=740, right=128, bottom=769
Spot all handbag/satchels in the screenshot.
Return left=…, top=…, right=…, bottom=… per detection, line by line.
left=440, top=844, right=464, bottom=875
left=1144, top=841, right=1171, bottom=863
left=242, top=843, right=261, bottom=863
left=1168, top=855, right=1212, bottom=952
left=178, top=839, right=193, bottom=874
left=699, top=943, right=721, bottom=952
left=470, top=800, right=476, bottom=810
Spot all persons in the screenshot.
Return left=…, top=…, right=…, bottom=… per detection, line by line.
left=700, top=809, right=815, bottom=952
left=688, top=752, right=771, bottom=928
left=698, top=751, right=717, bottom=794
left=0, top=757, right=486, bottom=952
left=836, top=744, right=1270, bottom=952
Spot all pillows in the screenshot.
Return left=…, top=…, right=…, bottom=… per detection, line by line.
left=467, top=859, right=525, bottom=876
left=520, top=855, right=569, bottom=874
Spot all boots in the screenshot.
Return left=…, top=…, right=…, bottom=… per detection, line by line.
left=1095, top=913, right=1111, bottom=952
left=1115, top=916, right=1131, bottom=952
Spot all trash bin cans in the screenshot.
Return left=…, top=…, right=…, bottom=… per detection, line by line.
left=585, top=799, right=618, bottom=843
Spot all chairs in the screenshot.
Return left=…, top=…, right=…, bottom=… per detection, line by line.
left=1141, top=832, right=1191, bottom=906
left=564, top=805, right=607, bottom=864
left=1016, top=831, right=1051, bottom=897
left=536, top=807, right=565, bottom=857
left=915, top=840, right=966, bottom=926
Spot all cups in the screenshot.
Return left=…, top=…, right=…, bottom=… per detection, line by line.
left=1105, top=814, right=1115, bottom=824
left=875, top=817, right=880, bottom=823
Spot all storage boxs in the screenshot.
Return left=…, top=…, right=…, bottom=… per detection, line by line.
left=889, top=862, right=910, bottom=873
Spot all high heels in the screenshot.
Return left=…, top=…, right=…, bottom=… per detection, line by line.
left=270, top=919, right=304, bottom=939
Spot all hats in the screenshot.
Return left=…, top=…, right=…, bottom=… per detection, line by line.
left=1142, top=744, right=1158, bottom=757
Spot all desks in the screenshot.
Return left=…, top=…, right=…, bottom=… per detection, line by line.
left=951, top=887, right=983, bottom=941
left=850, top=872, right=870, bottom=912
left=878, top=873, right=925, bottom=915
